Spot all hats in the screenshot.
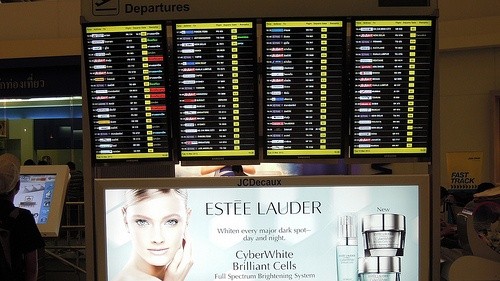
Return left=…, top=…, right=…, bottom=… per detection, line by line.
left=0, top=154, right=21, bottom=194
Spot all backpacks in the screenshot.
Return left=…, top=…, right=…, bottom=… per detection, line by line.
left=0, top=208, right=20, bottom=281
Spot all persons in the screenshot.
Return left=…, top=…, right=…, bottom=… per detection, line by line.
left=114, top=188, right=193, bottom=281
left=0, top=153, right=46, bottom=281
left=24, top=155, right=84, bottom=226
left=440, top=182, right=500, bottom=250
